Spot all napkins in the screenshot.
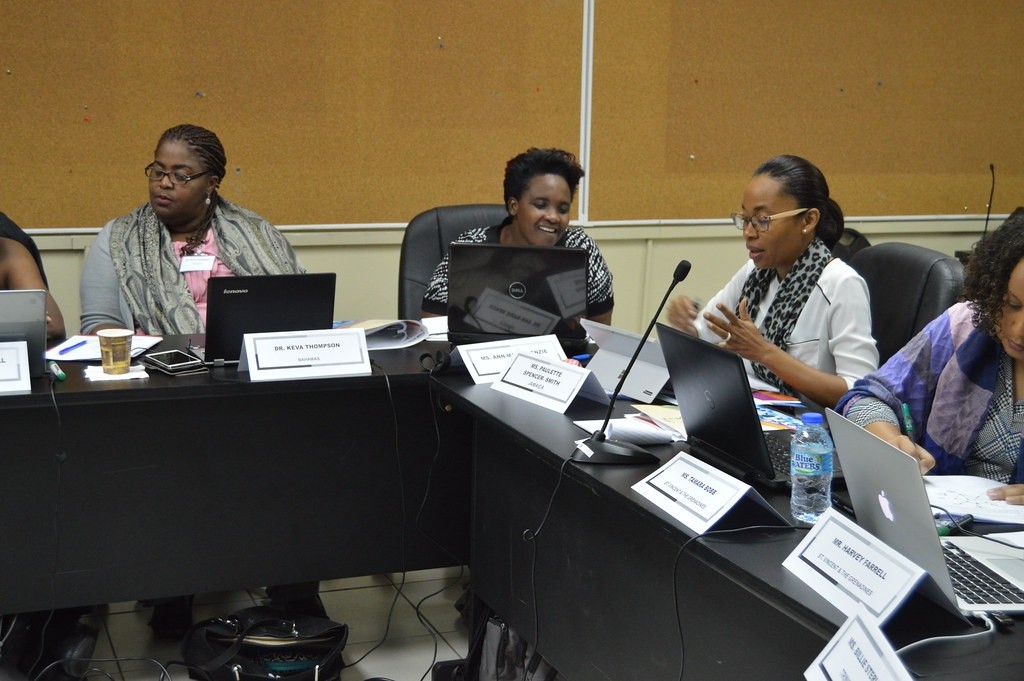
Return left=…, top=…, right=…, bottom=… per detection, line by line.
left=81, top=364, right=149, bottom=382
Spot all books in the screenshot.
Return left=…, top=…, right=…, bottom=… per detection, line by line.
left=333, top=319, right=427, bottom=351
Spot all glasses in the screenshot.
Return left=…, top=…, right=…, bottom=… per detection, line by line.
left=730, top=208, right=808, bottom=232
left=145, top=163, right=206, bottom=185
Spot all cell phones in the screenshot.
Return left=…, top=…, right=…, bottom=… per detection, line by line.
left=144, top=350, right=201, bottom=373
left=933, top=512, right=972, bottom=536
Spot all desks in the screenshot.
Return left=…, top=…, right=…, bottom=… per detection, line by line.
left=0, top=335, right=532, bottom=613
left=420, top=340, right=1023, bottom=681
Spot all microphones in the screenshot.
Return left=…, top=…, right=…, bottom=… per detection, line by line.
left=571, top=260, right=692, bottom=465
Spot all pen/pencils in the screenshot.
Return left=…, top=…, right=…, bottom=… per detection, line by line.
left=59, top=341, right=87, bottom=355
left=574, top=354, right=594, bottom=360
left=902, top=404, right=916, bottom=445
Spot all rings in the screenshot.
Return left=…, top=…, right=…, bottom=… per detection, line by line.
left=725, top=333, right=732, bottom=343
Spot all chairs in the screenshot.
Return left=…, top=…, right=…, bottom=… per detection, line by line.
left=397, top=205, right=509, bottom=328
left=848, top=242, right=967, bottom=367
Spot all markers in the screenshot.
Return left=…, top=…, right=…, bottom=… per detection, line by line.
left=48, top=361, right=66, bottom=381
left=938, top=514, right=974, bottom=536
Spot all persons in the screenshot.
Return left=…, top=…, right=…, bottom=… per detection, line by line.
left=665, top=155, right=882, bottom=412
left=79, top=124, right=302, bottom=620
left=419, top=148, right=615, bottom=327
left=0, top=235, right=67, bottom=343
left=832, top=205, right=1024, bottom=504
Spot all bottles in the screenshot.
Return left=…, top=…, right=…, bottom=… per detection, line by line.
left=788, top=411, right=834, bottom=525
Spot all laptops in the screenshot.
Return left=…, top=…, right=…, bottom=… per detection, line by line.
left=0, top=289, right=47, bottom=380
left=657, top=323, right=846, bottom=488
left=823, top=407, right=1024, bottom=616
left=444, top=240, right=591, bottom=350
left=186, top=272, right=338, bottom=368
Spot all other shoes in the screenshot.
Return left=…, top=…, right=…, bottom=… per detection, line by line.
left=56, top=622, right=99, bottom=676
left=149, top=597, right=194, bottom=643
left=269, top=583, right=327, bottom=623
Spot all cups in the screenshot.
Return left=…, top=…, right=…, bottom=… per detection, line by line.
left=97, top=328, right=135, bottom=375
